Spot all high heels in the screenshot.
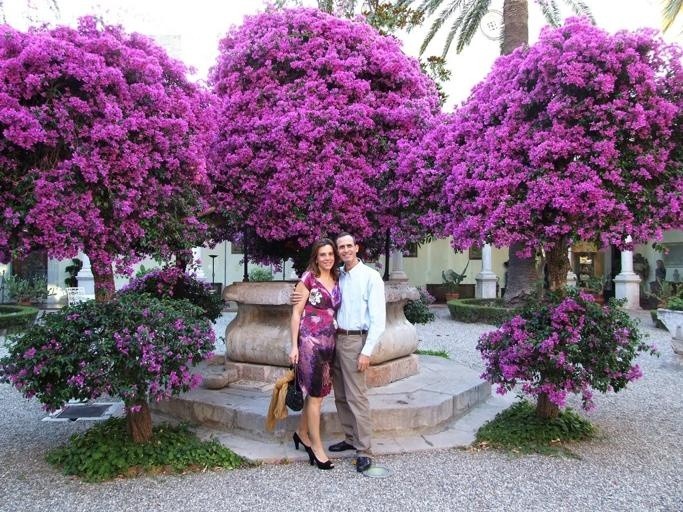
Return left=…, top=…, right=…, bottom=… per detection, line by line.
left=309, top=446, right=334, bottom=470
left=293, top=432, right=311, bottom=450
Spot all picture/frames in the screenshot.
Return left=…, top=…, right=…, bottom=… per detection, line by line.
left=402, top=245, right=418, bottom=257
left=469, top=246, right=482, bottom=260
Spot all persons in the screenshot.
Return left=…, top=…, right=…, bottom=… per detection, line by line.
left=289, top=231, right=386, bottom=473
left=287, top=237, right=342, bottom=470
left=495, top=251, right=666, bottom=308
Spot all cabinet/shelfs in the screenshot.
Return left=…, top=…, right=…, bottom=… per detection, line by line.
left=574, top=252, right=604, bottom=288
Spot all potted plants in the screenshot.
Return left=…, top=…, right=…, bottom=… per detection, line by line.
left=589, top=273, right=608, bottom=307
left=644, top=277, right=683, bottom=356
left=441, top=263, right=470, bottom=302
left=17, top=279, right=36, bottom=308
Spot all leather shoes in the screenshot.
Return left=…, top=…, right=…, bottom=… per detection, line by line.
left=357, top=457, right=372, bottom=472
left=329, top=441, right=357, bottom=452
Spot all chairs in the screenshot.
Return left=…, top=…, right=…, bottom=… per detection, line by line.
left=66, top=287, right=93, bottom=309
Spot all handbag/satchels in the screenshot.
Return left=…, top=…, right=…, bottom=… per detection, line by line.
left=285, top=360, right=304, bottom=412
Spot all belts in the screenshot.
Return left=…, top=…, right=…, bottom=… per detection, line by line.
left=338, top=328, right=368, bottom=335
